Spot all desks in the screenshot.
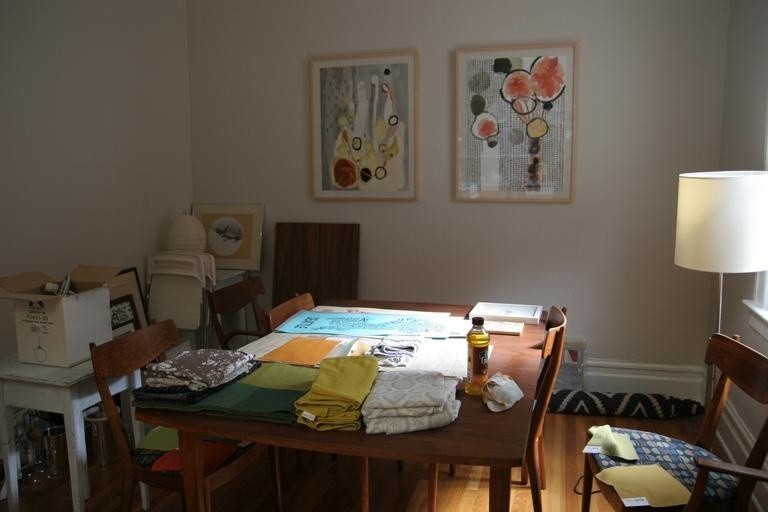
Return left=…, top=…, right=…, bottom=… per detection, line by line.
left=0, top=359, right=155, bottom=512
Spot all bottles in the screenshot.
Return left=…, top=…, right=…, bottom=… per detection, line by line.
left=467, top=316, right=490, bottom=398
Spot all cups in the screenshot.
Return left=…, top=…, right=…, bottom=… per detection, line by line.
left=45, top=427, right=69, bottom=476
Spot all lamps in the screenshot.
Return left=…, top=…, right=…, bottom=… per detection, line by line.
left=673, top=169, right=768, bottom=412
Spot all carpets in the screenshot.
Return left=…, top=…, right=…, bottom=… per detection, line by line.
left=549, top=389, right=705, bottom=419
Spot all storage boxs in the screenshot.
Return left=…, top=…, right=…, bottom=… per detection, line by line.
left=0, top=263, right=121, bottom=368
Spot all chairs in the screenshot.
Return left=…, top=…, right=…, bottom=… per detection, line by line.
left=89, top=277, right=567, bottom=512
left=582, top=333, right=768, bottom=512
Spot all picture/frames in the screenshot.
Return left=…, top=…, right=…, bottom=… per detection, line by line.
left=308, top=48, right=420, bottom=201
left=191, top=203, right=264, bottom=271
left=453, top=42, right=578, bottom=204
